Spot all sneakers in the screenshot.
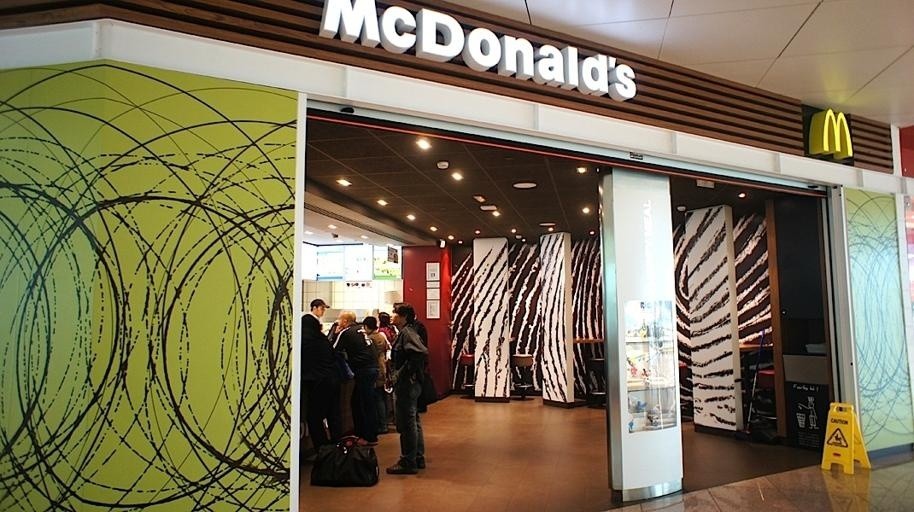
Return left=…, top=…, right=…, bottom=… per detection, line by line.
left=386, top=455, right=425, bottom=474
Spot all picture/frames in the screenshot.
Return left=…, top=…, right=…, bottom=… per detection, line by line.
left=426, top=262, right=440, bottom=319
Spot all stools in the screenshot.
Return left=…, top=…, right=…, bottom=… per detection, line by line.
left=511, top=353, right=536, bottom=402
left=744, top=371, right=781, bottom=434
left=460, top=354, right=475, bottom=399
left=588, top=355, right=608, bottom=405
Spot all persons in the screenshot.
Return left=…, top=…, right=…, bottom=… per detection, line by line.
left=384, top=305, right=430, bottom=474
left=333, top=310, right=381, bottom=448
left=301, top=299, right=429, bottom=463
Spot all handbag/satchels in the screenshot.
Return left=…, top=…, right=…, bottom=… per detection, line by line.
left=310, top=435, right=379, bottom=487
left=419, top=370, right=438, bottom=406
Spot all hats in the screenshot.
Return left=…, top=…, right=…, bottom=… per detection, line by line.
left=310, top=299, right=329, bottom=308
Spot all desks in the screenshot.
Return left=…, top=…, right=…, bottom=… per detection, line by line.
left=739, top=343, right=775, bottom=423
left=573, top=339, right=603, bottom=399
